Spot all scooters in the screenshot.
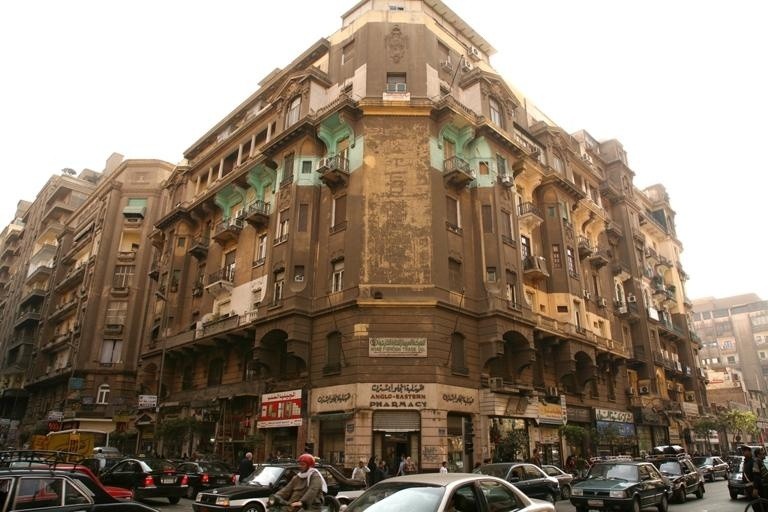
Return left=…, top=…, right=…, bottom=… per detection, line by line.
left=265, top=480, right=350, bottom=512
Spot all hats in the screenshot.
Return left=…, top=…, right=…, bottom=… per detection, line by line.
left=740, top=445, right=751, bottom=451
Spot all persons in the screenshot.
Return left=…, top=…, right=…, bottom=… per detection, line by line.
left=239, top=451, right=492, bottom=512
left=577, top=454, right=589, bottom=478
left=181, top=453, right=189, bottom=460
left=640, top=444, right=768, bottom=512
left=530, top=449, right=542, bottom=468
left=94, top=449, right=106, bottom=471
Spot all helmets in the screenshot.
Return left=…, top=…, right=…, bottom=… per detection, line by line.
left=297, top=454, right=316, bottom=469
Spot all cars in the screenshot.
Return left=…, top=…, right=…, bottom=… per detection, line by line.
left=0, top=446, right=160, bottom=512
left=727, top=455, right=768, bottom=500
left=569, top=460, right=674, bottom=512
left=191, top=462, right=369, bottom=512
left=645, top=445, right=706, bottom=505
left=691, top=456, right=731, bottom=482
left=345, top=472, right=556, bottom=512
left=95, top=457, right=190, bottom=504
left=175, top=460, right=236, bottom=500
left=471, top=462, right=561, bottom=507
left=539, top=465, right=575, bottom=501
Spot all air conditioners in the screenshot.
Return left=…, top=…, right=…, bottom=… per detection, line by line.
left=732, top=382, right=740, bottom=387
left=236, top=208, right=244, bottom=221
left=488, top=377, right=504, bottom=389
left=583, top=289, right=636, bottom=308
left=640, top=386, right=649, bottom=394
left=550, top=386, right=558, bottom=397
left=583, top=152, right=592, bottom=166
left=667, top=380, right=683, bottom=393
left=316, top=158, right=328, bottom=173
left=441, top=46, right=480, bottom=74
left=503, top=174, right=513, bottom=186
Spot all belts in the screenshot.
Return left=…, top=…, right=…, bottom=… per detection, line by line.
left=745, top=482, right=754, bottom=486
